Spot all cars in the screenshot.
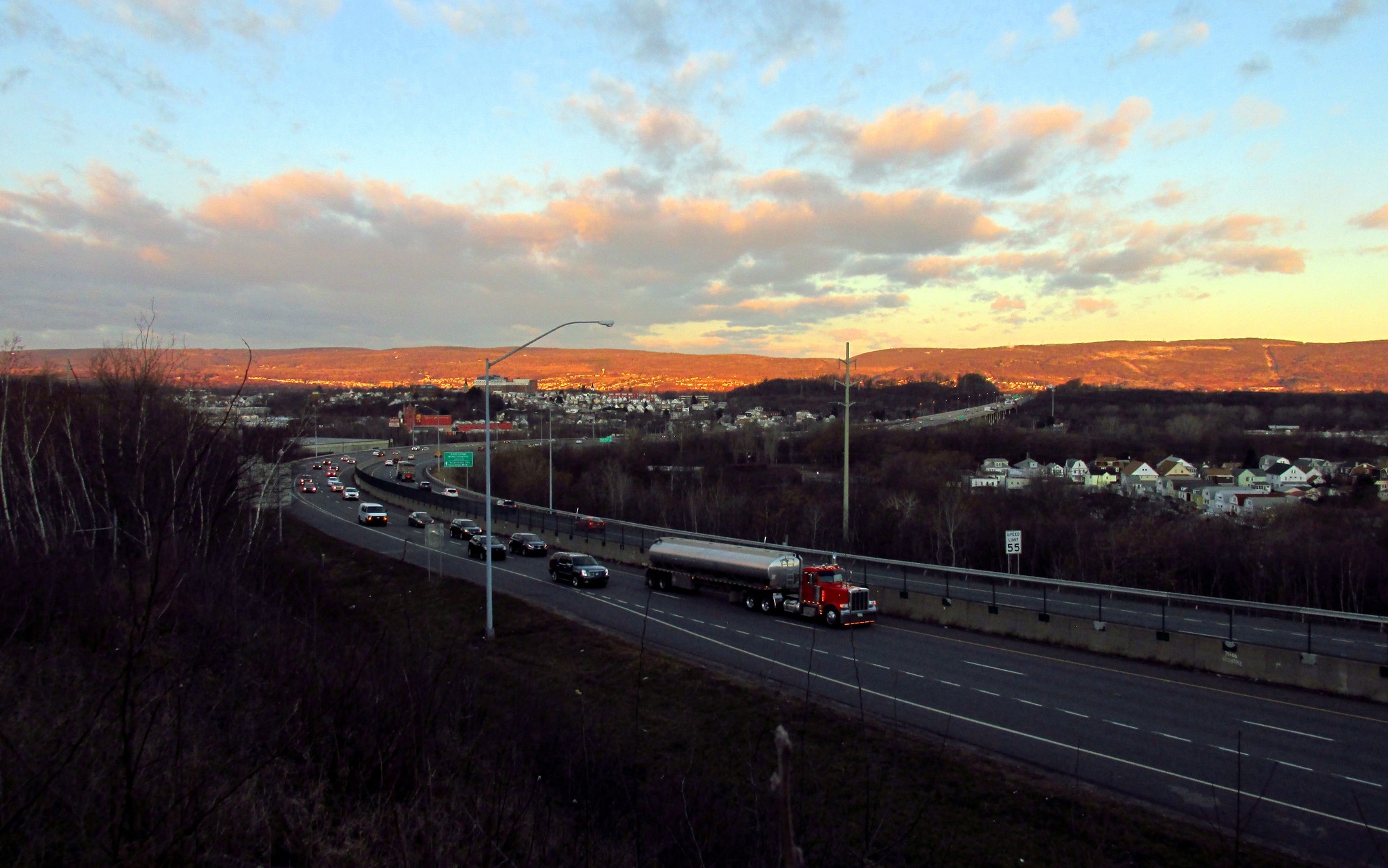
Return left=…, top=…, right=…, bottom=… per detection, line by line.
left=327, top=477, right=340, bottom=487
left=312, top=455, right=357, bottom=477
left=330, top=482, right=345, bottom=492
left=373, top=445, right=430, bottom=467
left=574, top=516, right=605, bottom=533
left=477, top=438, right=559, bottom=452
left=408, top=512, right=434, bottom=528
left=302, top=482, right=316, bottom=494
left=342, top=487, right=359, bottom=501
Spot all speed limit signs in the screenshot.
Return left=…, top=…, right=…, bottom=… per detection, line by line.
left=1006, top=530, right=1022, bottom=554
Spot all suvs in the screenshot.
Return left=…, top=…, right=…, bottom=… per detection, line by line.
left=449, top=519, right=482, bottom=541
left=508, top=533, right=548, bottom=557
left=441, top=488, right=459, bottom=500
left=299, top=474, right=314, bottom=486
left=548, top=551, right=610, bottom=588
left=468, top=535, right=507, bottom=561
left=417, top=481, right=432, bottom=493
left=495, top=499, right=517, bottom=514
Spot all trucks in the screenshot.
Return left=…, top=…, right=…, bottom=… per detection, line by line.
left=395, top=461, right=416, bottom=483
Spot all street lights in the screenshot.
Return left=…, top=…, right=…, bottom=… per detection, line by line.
left=484, top=320, right=615, bottom=639
left=424, top=405, right=440, bottom=473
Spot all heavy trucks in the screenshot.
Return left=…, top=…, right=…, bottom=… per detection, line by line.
left=641, top=536, right=877, bottom=630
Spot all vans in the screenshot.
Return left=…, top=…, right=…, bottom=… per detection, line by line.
left=358, top=502, right=388, bottom=527
